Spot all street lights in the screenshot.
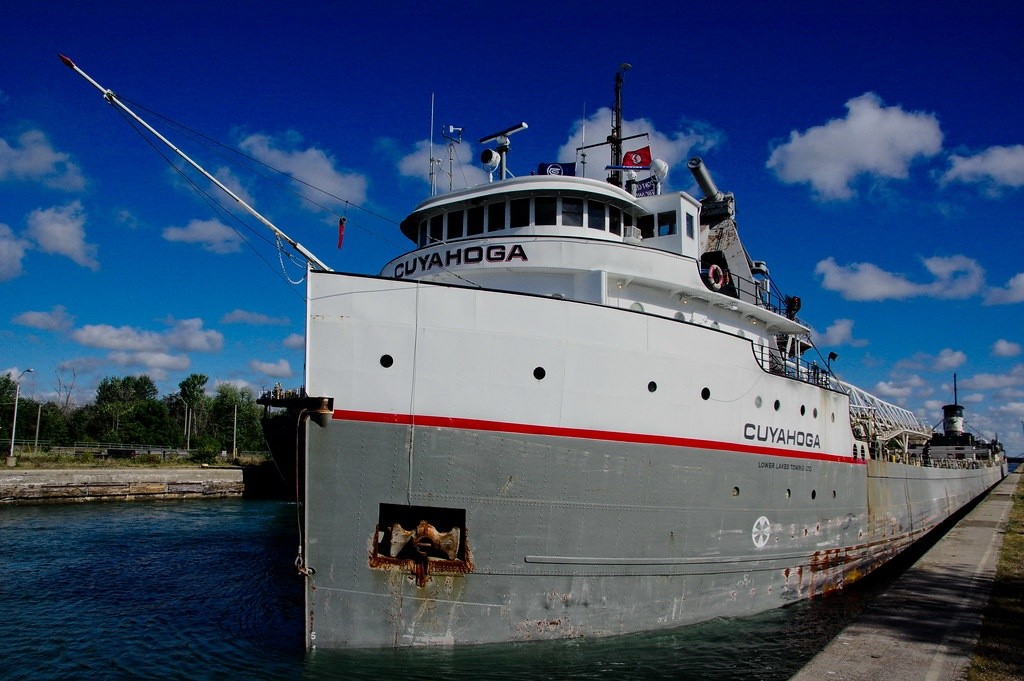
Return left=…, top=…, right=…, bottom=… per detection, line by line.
left=7, top=366, right=34, bottom=457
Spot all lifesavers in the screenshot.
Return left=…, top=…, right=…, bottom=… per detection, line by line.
left=708, top=263, right=725, bottom=291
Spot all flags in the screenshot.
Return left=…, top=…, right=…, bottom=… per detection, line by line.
left=538, top=162, right=576, bottom=176
left=626, top=175, right=661, bottom=197
left=622, top=146, right=651, bottom=172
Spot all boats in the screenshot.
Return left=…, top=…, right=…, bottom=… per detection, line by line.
left=48, top=48, right=1009, bottom=661
